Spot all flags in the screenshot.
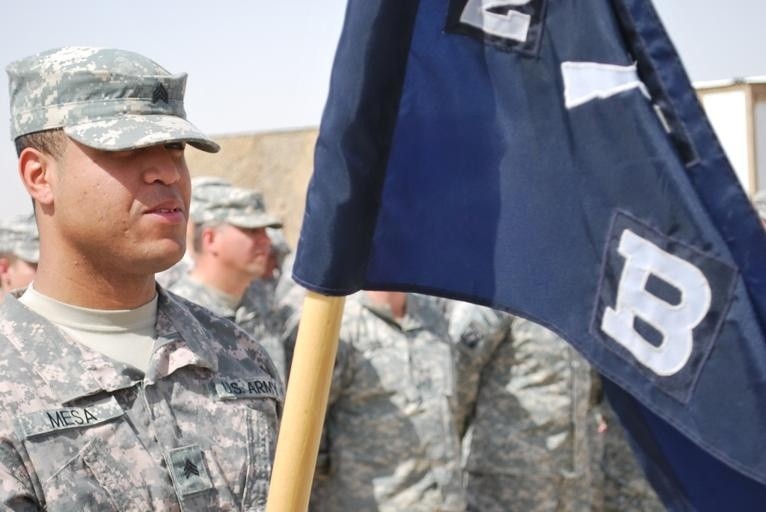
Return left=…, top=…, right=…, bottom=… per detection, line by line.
left=291, top=0, right=766, bottom=510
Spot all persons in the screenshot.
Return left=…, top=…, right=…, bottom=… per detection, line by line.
left=0, top=40, right=285, bottom=511
left=2, top=174, right=666, bottom=508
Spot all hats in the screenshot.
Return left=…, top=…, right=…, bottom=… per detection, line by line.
left=186, top=177, right=284, bottom=229
left=5, top=47, right=222, bottom=154
left=0, top=215, right=41, bottom=266
